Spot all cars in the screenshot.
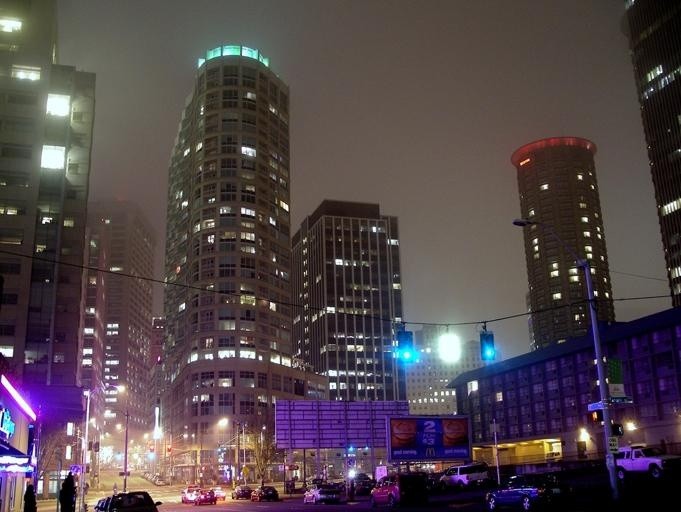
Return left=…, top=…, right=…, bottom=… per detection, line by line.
left=485, top=469, right=574, bottom=511
left=91, top=471, right=280, bottom=512
left=370, top=471, right=428, bottom=508
left=303, top=484, right=343, bottom=504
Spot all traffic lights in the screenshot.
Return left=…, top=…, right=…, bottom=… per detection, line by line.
left=480, top=330, right=495, bottom=361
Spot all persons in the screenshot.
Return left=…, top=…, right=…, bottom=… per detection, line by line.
left=59, top=471, right=75, bottom=512
left=24, top=485, right=37, bottom=512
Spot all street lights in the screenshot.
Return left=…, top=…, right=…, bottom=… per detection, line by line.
left=511, top=217, right=620, bottom=504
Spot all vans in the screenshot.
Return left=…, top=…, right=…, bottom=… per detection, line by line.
left=438, top=464, right=489, bottom=490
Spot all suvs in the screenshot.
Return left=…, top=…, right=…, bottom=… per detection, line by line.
left=615, top=446, right=681, bottom=479
